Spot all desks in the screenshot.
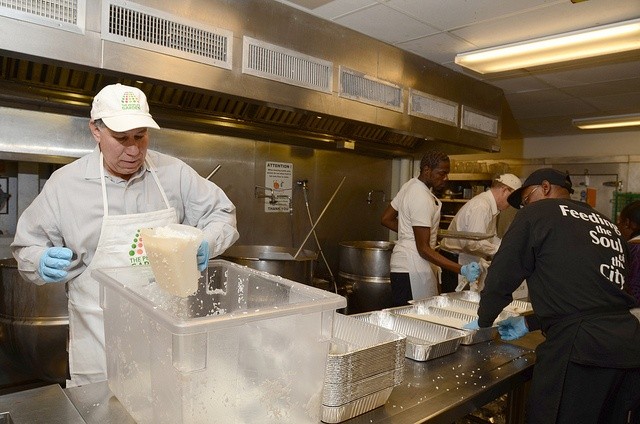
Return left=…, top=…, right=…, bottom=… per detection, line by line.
left=0, top=383, right=90, bottom=424
left=61, top=341, right=538, bottom=424
left=500, top=296, right=548, bottom=350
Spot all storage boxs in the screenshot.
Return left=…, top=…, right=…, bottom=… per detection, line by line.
left=90, top=259, right=348, bottom=424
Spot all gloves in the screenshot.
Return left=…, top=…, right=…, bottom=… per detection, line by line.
left=37, top=246, right=73, bottom=283
left=197, top=240, right=209, bottom=271
left=463, top=319, right=480, bottom=329
left=496, top=315, right=529, bottom=340
left=460, top=261, right=480, bottom=283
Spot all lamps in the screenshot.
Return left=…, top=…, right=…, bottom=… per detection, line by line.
left=577, top=120, right=640, bottom=130
left=455, top=16, right=640, bottom=74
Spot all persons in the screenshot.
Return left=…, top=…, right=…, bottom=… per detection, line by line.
left=616, top=200, right=640, bottom=307
left=9, top=83, right=241, bottom=387
left=438, top=174, right=523, bottom=291
left=381, top=148, right=479, bottom=308
left=477, top=168, right=640, bottom=423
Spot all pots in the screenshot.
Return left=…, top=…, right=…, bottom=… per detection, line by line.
left=336, top=240, right=393, bottom=281
left=225, top=245, right=318, bottom=290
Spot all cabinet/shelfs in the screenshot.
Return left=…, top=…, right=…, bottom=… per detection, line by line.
left=430, top=159, right=550, bottom=245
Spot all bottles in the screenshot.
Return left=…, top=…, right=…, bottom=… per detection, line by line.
left=461, top=181, right=472, bottom=199
left=578, top=182, right=585, bottom=202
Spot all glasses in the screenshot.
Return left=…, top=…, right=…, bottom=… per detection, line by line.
left=519, top=187, right=541, bottom=208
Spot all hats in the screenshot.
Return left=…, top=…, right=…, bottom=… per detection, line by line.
left=495, top=173, right=523, bottom=190
left=90, top=83, right=160, bottom=133
left=507, top=168, right=574, bottom=209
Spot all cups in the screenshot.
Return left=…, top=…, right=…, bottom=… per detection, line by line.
left=453, top=162, right=488, bottom=173
left=586, top=188, right=596, bottom=208
left=489, top=162, right=509, bottom=175
left=141, top=224, right=204, bottom=298
